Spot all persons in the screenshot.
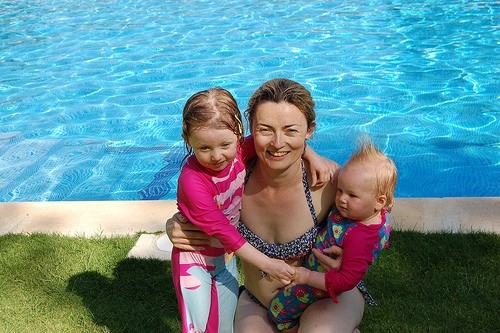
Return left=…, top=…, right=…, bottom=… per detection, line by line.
left=267, top=134, right=398, bottom=333
left=171, top=85, right=336, bottom=333
left=165, top=78, right=342, bottom=333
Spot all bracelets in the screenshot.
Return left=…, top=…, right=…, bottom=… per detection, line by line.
left=302, top=140, right=306, bottom=158
